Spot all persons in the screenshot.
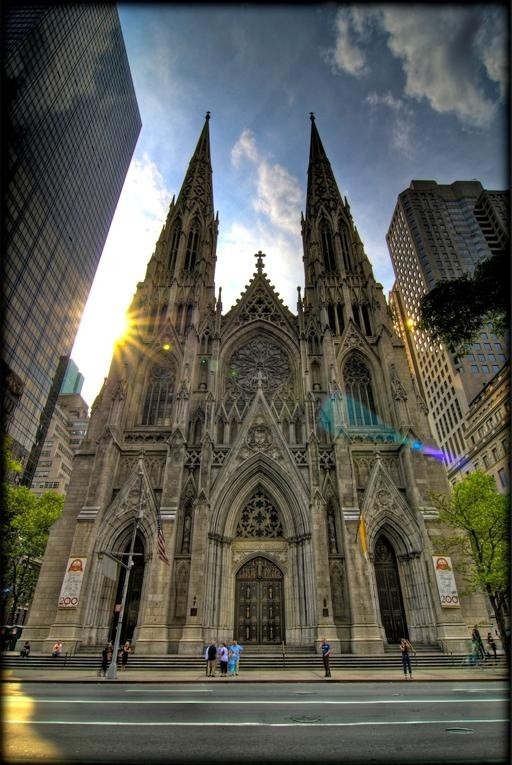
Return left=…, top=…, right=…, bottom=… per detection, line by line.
left=205, top=640, right=244, bottom=677
left=97, top=643, right=112, bottom=677
left=21, top=642, right=30, bottom=658
left=52, top=640, right=62, bottom=656
left=122, top=641, right=131, bottom=670
left=400, top=640, right=413, bottom=680
left=472, top=625, right=485, bottom=658
left=488, top=633, right=497, bottom=658
left=319, top=638, right=331, bottom=677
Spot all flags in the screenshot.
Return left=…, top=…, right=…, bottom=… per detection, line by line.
left=157, top=520, right=170, bottom=566
left=359, top=514, right=369, bottom=560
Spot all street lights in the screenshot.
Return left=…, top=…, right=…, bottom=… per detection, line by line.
left=105, top=461, right=150, bottom=679
